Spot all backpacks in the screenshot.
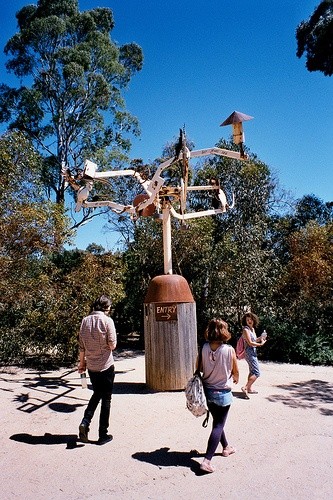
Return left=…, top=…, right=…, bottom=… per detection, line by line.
left=185, top=345, right=209, bottom=418
left=235, top=335, right=246, bottom=359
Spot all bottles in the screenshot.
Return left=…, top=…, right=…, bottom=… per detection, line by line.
left=261, top=330, right=267, bottom=341
left=80, top=371, right=88, bottom=389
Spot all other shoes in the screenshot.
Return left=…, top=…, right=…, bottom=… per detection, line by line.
left=79, top=424, right=90, bottom=442
left=242, top=386, right=250, bottom=400
left=223, top=446, right=236, bottom=456
left=200, top=462, right=216, bottom=472
left=249, top=390, right=258, bottom=394
left=99, top=433, right=112, bottom=444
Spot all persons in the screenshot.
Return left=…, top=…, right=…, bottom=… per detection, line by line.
left=196, top=318, right=239, bottom=472
left=78, top=295, right=117, bottom=445
left=241, top=313, right=267, bottom=399
